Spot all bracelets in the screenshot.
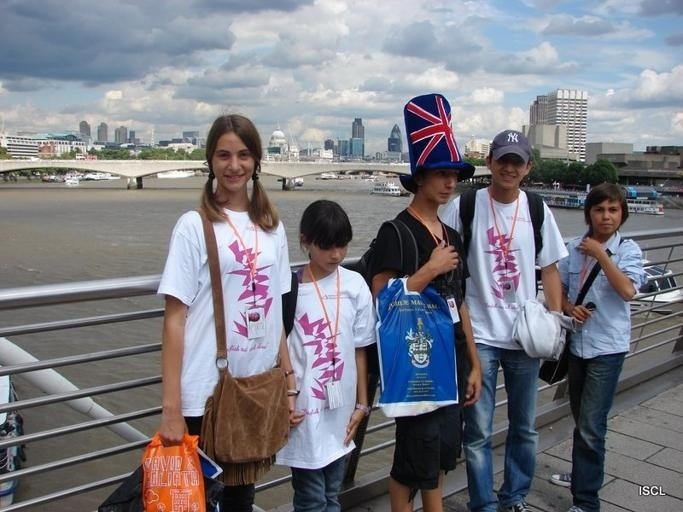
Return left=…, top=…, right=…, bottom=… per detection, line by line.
left=354, top=402, right=371, bottom=417
left=285, top=388, right=300, bottom=394
left=281, top=368, right=295, bottom=376
left=286, top=393, right=297, bottom=397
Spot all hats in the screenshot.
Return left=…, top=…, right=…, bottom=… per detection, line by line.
left=490, top=129, right=532, bottom=164
left=399, top=93, right=475, bottom=193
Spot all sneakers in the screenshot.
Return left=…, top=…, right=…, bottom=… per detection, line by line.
left=549, top=473, right=571, bottom=487
left=499, top=502, right=532, bottom=512
left=565, top=506, right=586, bottom=512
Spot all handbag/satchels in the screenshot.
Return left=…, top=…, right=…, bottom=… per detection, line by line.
left=199, top=366, right=290, bottom=487
left=97, top=464, right=223, bottom=512
left=537, top=328, right=574, bottom=384
left=374, top=275, right=458, bottom=418
left=510, top=299, right=560, bottom=359
left=142, top=431, right=206, bottom=511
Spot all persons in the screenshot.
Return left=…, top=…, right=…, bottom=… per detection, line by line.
left=438, top=128, right=570, bottom=509
left=272, top=199, right=377, bottom=510
left=355, top=166, right=484, bottom=511
left=153, top=111, right=307, bottom=511
left=550, top=180, right=649, bottom=509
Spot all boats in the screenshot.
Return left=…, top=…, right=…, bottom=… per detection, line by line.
left=369, top=181, right=410, bottom=197
left=156, top=170, right=196, bottom=179
left=626, top=198, right=665, bottom=215
left=632, top=259, right=683, bottom=302
left=527, top=189, right=587, bottom=207
left=0, top=365, right=25, bottom=510
left=42, top=169, right=121, bottom=187
left=316, top=172, right=375, bottom=179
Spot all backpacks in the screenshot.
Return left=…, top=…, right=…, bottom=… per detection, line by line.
left=356, top=219, right=462, bottom=289
